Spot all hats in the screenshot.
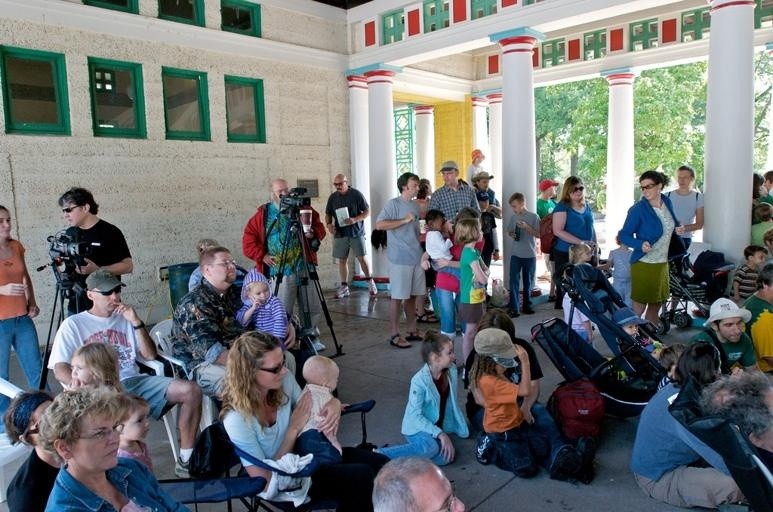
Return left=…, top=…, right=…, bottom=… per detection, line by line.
left=611, top=307, right=650, bottom=327
left=475, top=328, right=519, bottom=360
left=85, top=269, right=126, bottom=295
left=471, top=148, right=486, bottom=164
left=476, top=191, right=490, bottom=201
left=539, top=178, right=560, bottom=191
left=471, top=171, right=494, bottom=181
left=438, top=161, right=458, bottom=174
left=702, top=297, right=752, bottom=327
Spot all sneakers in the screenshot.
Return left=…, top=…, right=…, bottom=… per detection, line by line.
left=550, top=445, right=576, bottom=481
left=576, top=436, right=596, bottom=484
left=175, top=455, right=197, bottom=478
left=509, top=310, right=520, bottom=318
left=368, top=278, right=378, bottom=295
left=334, top=285, right=350, bottom=299
left=313, top=342, right=327, bottom=352
left=523, top=307, right=535, bottom=314
left=510, top=312, right=521, bottom=318
left=474, top=433, right=492, bottom=464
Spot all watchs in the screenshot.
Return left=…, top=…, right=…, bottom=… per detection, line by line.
left=132, top=322, right=145, bottom=332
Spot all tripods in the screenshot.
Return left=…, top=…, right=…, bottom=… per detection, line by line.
left=35, top=235, right=96, bottom=395
left=263, top=189, right=346, bottom=360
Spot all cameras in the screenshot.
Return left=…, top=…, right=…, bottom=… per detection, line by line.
left=278, top=188, right=312, bottom=220
left=46, top=232, right=94, bottom=276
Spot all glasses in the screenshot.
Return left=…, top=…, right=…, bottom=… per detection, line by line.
left=333, top=182, right=343, bottom=186
left=572, top=186, right=584, bottom=194
left=207, top=259, right=236, bottom=267
left=639, top=184, right=657, bottom=191
left=62, top=205, right=81, bottom=213
left=72, top=423, right=126, bottom=441
left=256, top=359, right=288, bottom=374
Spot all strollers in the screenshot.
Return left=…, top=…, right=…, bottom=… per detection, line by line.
left=654, top=250, right=735, bottom=333
left=531, top=262, right=668, bottom=420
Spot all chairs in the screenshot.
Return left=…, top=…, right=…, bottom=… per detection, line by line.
left=53, top=352, right=182, bottom=465
left=214, top=398, right=377, bottom=512
left=147, top=318, right=217, bottom=433
left=1, top=375, right=33, bottom=512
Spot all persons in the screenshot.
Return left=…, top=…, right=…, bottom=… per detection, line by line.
left=37, top=389, right=187, bottom=512
left=372, top=454, right=464, bottom=512
left=474, top=190, right=500, bottom=276
left=763, top=230, right=773, bottom=262
left=660, top=166, right=704, bottom=328
left=374, top=172, right=426, bottom=349
left=52, top=187, right=134, bottom=319
left=273, top=356, right=343, bottom=477
left=505, top=193, right=541, bottom=318
left=188, top=237, right=244, bottom=294
left=243, top=178, right=327, bottom=351
left=658, top=344, right=688, bottom=392
left=472, top=173, right=500, bottom=220
left=65, top=343, right=123, bottom=392
left=751, top=203, right=773, bottom=246
left=434, top=206, right=484, bottom=343
left=454, top=218, right=490, bottom=388
left=630, top=340, right=748, bottom=512
left=372, top=329, right=470, bottom=466
left=537, top=177, right=558, bottom=302
left=116, top=393, right=155, bottom=479
left=729, top=246, right=767, bottom=307
left=171, top=247, right=303, bottom=415
left=46, top=269, right=202, bottom=481
left=6, top=392, right=55, bottom=512
left=610, top=307, right=666, bottom=363
left=413, top=177, right=432, bottom=253
left=752, top=171, right=769, bottom=225
left=325, top=175, right=377, bottom=299
left=763, top=170, right=773, bottom=195
left=704, top=371, right=773, bottom=463
left=621, top=170, right=685, bottom=331
left=425, top=209, right=461, bottom=284
left=468, top=328, right=550, bottom=479
left=428, top=159, right=482, bottom=238
left=549, top=176, right=599, bottom=299
left=468, top=149, right=485, bottom=190
left=236, top=273, right=287, bottom=347
left=594, top=230, right=636, bottom=314
left=463, top=307, right=596, bottom=486
left=0, top=205, right=52, bottom=437
left=566, top=242, right=593, bottom=273
left=555, top=264, right=594, bottom=353
left=219, top=331, right=391, bottom=512
left=739, top=263, right=773, bottom=379
left=688, top=297, right=763, bottom=379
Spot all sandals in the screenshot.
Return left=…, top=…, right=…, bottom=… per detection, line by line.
left=415, top=313, right=440, bottom=323
left=389, top=335, right=412, bottom=348
left=422, top=309, right=435, bottom=315
left=405, top=329, right=425, bottom=341
